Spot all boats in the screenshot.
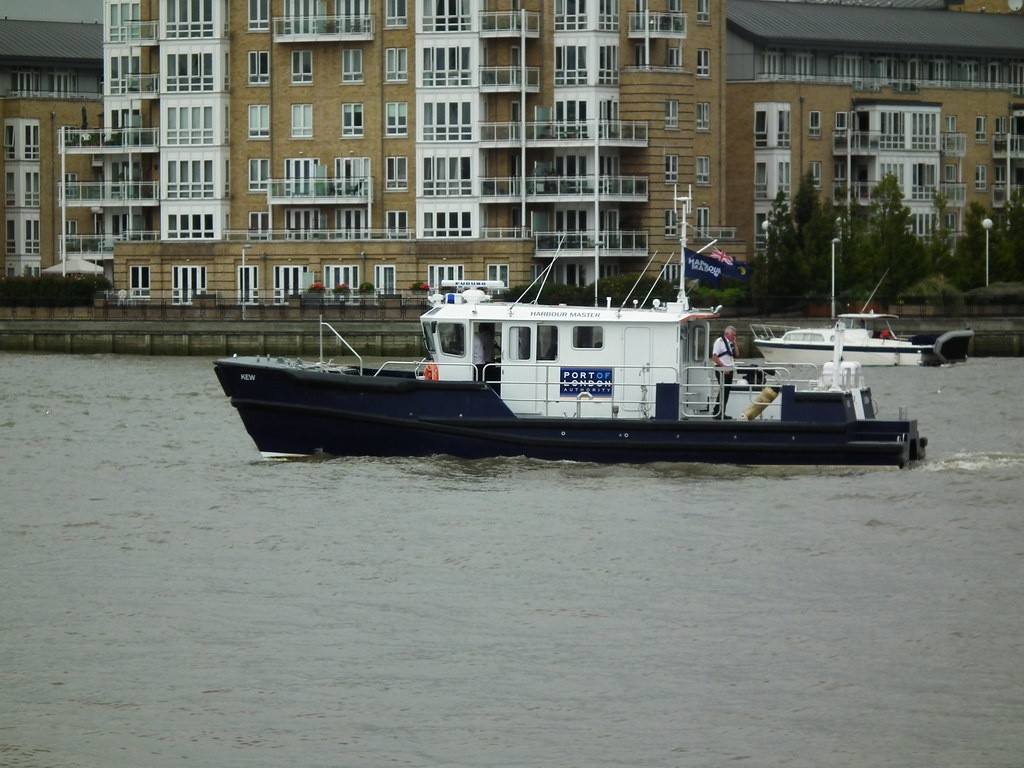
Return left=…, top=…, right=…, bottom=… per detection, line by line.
left=212, top=185, right=932, bottom=472
left=748, top=268, right=944, bottom=369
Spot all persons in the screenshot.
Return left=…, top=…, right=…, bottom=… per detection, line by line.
left=713, top=326, right=740, bottom=419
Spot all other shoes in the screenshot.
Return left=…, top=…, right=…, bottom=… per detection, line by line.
left=713, top=412, right=732, bottom=420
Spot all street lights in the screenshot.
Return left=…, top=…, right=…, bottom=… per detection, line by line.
left=828, top=236, right=841, bottom=330
left=983, top=218, right=994, bottom=290
left=241, top=244, right=252, bottom=322
left=761, top=217, right=771, bottom=269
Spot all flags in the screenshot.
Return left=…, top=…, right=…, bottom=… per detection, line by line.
left=684, top=247, right=723, bottom=287
left=711, top=245, right=751, bottom=281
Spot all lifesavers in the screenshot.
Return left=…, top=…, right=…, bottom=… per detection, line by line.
left=423, top=362, right=439, bottom=381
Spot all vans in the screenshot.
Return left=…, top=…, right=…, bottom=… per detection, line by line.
left=438, top=279, right=505, bottom=306
